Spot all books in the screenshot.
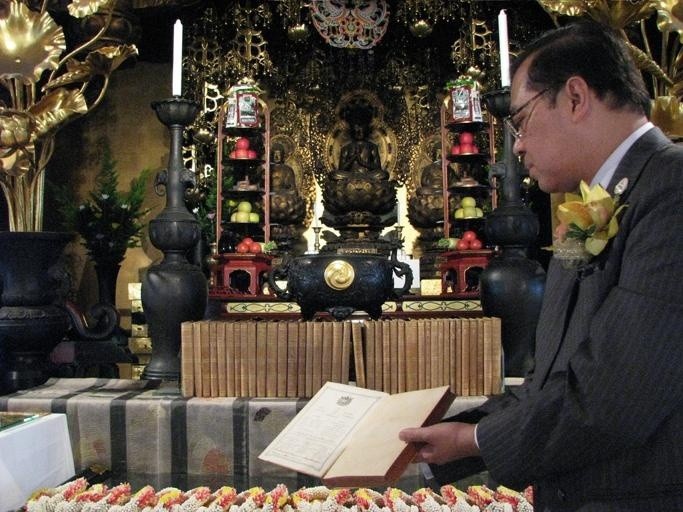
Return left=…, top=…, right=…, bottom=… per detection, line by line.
left=254, top=378, right=457, bottom=488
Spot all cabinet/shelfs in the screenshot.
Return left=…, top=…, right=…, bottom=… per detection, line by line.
left=215, top=96, right=272, bottom=253
left=440, top=87, right=498, bottom=253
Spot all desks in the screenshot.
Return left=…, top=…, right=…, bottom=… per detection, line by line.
left=0, top=374, right=497, bottom=495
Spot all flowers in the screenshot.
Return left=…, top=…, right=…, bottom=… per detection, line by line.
left=44, top=141, right=162, bottom=267
left=536, top=0, right=683, bottom=141
left=539, top=178, right=632, bottom=263
left=0, top=0, right=139, bottom=234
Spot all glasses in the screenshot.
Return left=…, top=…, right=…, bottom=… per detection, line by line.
left=502, top=83, right=559, bottom=139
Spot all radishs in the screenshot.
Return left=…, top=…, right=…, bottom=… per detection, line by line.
left=437, top=237, right=461, bottom=249
left=255, top=241, right=278, bottom=255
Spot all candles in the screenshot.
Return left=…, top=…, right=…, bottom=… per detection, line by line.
left=171, top=16, right=184, bottom=99
left=496, top=7, right=512, bottom=90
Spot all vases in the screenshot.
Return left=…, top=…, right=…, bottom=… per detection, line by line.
left=0, top=229, right=79, bottom=390
left=94, top=264, right=121, bottom=308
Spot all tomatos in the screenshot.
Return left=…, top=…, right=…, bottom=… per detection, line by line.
left=456, top=232, right=483, bottom=250
left=236, top=236, right=261, bottom=255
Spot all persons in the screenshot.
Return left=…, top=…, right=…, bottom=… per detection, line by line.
left=413, top=143, right=458, bottom=195
left=398, top=17, right=682, bottom=510
left=329, top=112, right=389, bottom=182
left=259, top=144, right=295, bottom=193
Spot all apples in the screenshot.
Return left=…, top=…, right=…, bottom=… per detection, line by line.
left=231, top=201, right=260, bottom=224
left=450, top=133, right=478, bottom=155
left=454, top=196, right=483, bottom=218
left=228, top=137, right=257, bottom=160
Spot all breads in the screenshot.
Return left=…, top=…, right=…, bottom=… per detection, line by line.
left=232, top=180, right=258, bottom=192
left=462, top=174, right=476, bottom=185
